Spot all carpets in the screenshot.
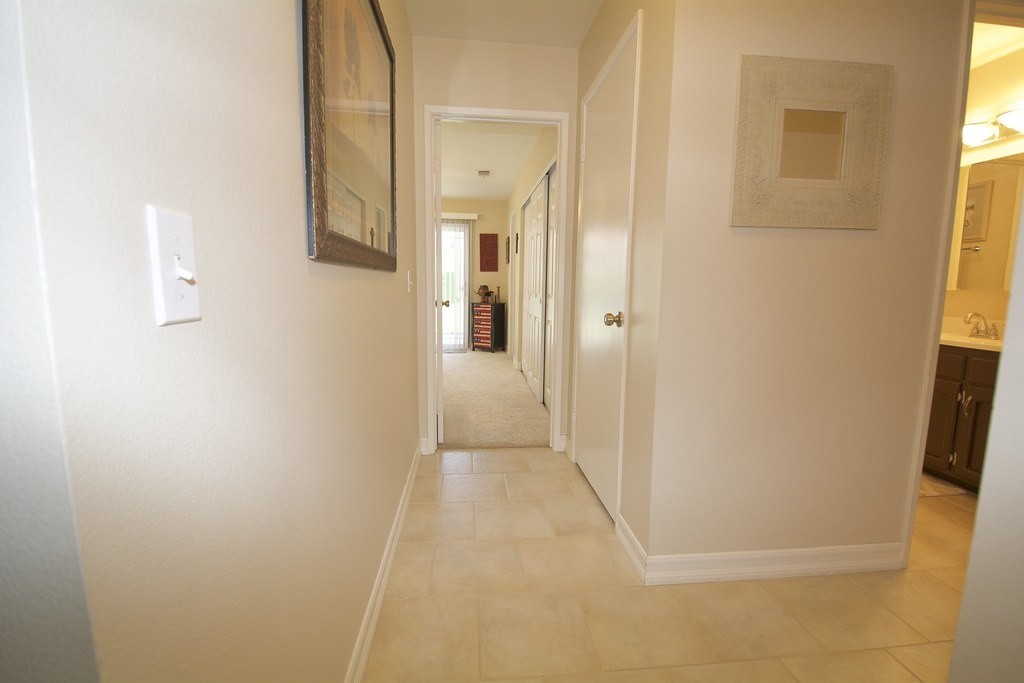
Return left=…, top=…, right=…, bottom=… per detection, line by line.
left=919, top=471, right=969, bottom=498
left=435, top=350, right=550, bottom=448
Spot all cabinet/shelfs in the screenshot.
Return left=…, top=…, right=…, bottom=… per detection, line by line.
left=924, top=344, right=1001, bottom=493
left=471, top=302, right=505, bottom=353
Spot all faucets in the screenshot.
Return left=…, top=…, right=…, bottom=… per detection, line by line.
left=963, top=312, right=989, bottom=335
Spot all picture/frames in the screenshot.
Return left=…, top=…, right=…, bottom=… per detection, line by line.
left=729, top=53, right=896, bottom=229
left=962, top=180, right=994, bottom=242
left=302, top=0, right=399, bottom=272
left=505, top=236, right=509, bottom=263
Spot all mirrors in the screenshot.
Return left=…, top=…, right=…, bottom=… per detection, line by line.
left=948, top=151, right=1024, bottom=293
